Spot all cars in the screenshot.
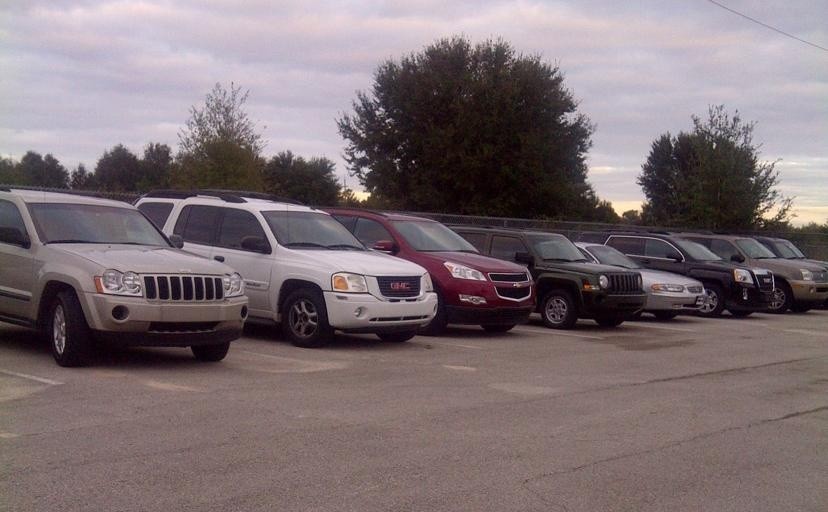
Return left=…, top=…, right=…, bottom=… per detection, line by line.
left=540, top=240, right=706, bottom=323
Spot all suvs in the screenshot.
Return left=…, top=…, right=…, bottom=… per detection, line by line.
left=445, top=223, right=647, bottom=331
left=599, top=228, right=826, bottom=319
left=309, top=205, right=537, bottom=337
left=130, top=192, right=440, bottom=349
left=2, top=190, right=251, bottom=362
